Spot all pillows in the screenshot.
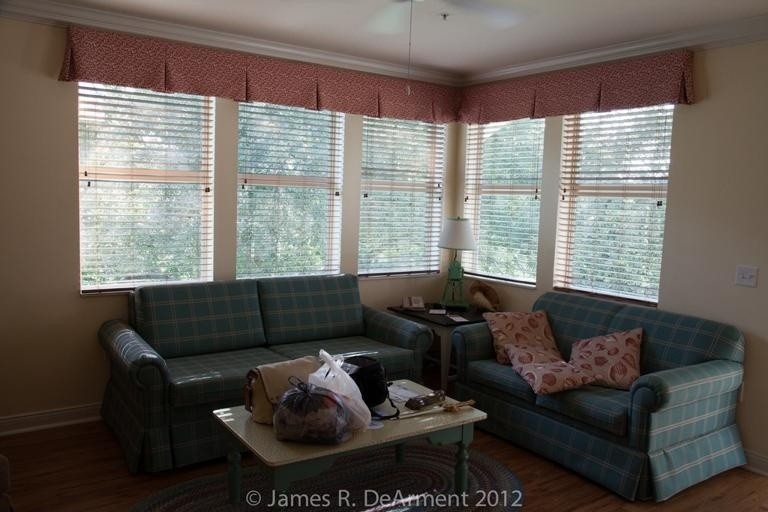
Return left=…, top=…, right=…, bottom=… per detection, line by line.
left=504, top=344, right=595, bottom=396
left=570, top=327, right=645, bottom=390
left=482, top=311, right=562, bottom=364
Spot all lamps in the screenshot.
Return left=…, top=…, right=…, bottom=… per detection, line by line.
left=437, top=217, right=477, bottom=310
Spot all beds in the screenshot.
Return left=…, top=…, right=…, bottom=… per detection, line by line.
left=211, top=379, right=489, bottom=505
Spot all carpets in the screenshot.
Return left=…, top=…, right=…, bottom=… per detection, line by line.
left=127, top=440, right=526, bottom=512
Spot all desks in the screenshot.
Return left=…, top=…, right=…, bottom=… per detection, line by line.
left=386, top=304, right=495, bottom=397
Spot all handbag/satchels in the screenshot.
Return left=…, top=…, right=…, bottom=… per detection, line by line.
left=243, top=356, right=320, bottom=425
left=341, top=355, right=400, bottom=419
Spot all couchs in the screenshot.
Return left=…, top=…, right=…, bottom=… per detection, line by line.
left=98, top=273, right=434, bottom=476
left=449, top=290, right=748, bottom=503
